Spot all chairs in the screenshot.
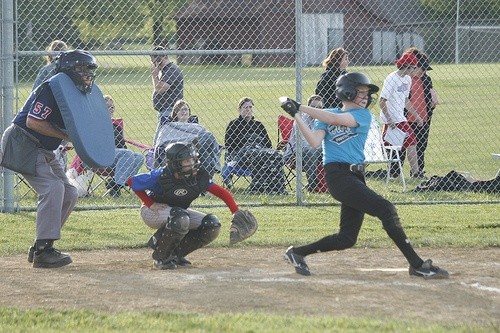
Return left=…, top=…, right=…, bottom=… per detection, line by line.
left=86, top=118, right=132, bottom=197
left=225, top=146, right=254, bottom=190
left=364, top=114, right=407, bottom=194
left=278, top=118, right=306, bottom=191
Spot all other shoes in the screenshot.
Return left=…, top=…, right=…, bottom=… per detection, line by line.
left=180, top=260, right=191, bottom=266
left=152, top=261, right=178, bottom=271
left=105, top=179, right=122, bottom=197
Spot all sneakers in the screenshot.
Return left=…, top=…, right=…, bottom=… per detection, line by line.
left=26, top=247, right=35, bottom=262
left=284, top=246, right=311, bottom=276
left=408, top=258, right=449, bottom=279
left=32, top=244, right=73, bottom=268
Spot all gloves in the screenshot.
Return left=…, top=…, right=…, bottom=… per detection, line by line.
left=280, top=97, right=301, bottom=118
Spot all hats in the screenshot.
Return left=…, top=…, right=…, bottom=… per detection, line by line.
left=416, top=53, right=433, bottom=71
left=395, top=54, right=418, bottom=69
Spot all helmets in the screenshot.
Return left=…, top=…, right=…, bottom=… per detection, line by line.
left=55, top=49, right=98, bottom=94
left=334, top=72, right=380, bottom=108
left=164, top=142, right=199, bottom=173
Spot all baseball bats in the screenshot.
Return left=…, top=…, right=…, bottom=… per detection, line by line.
left=279, top=97, right=291, bottom=109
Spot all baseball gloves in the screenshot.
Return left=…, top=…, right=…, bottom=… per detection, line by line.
left=230, top=210, right=258, bottom=245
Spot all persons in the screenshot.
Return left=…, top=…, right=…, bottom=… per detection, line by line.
left=128, top=142, right=258, bottom=270
left=315, top=48, right=350, bottom=109
left=85, top=95, right=144, bottom=199
left=378, top=48, right=439, bottom=181
left=286, top=94, right=325, bottom=193
left=225, top=98, right=287, bottom=195
left=0, top=49, right=99, bottom=268
left=154, top=100, right=221, bottom=197
left=279, top=73, right=450, bottom=280
left=32, top=41, right=67, bottom=173
left=149, top=46, right=184, bottom=146
